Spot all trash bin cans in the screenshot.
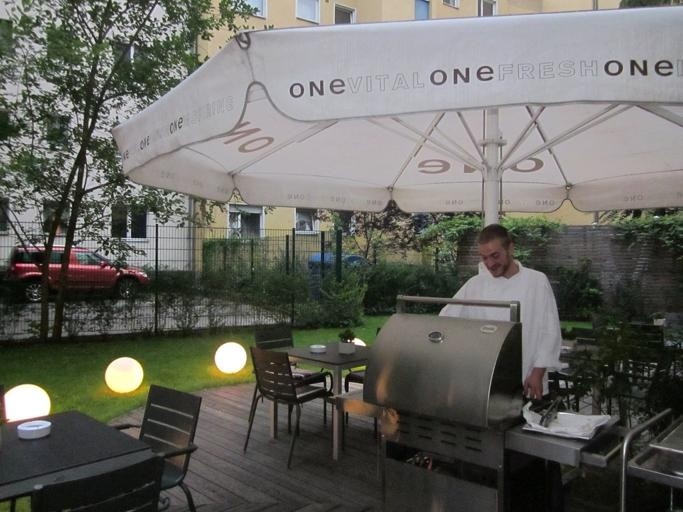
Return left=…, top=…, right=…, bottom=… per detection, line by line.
left=308, top=253, right=363, bottom=299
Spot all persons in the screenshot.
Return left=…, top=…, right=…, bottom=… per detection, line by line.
left=436, top=223, right=563, bottom=402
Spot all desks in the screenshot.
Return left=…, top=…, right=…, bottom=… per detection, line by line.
left=2, top=409, right=150, bottom=508
left=280, top=343, right=379, bottom=460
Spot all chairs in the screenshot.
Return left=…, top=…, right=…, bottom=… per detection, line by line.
left=621, top=322, right=680, bottom=378
left=28, top=385, right=200, bottom=512
left=241, top=322, right=332, bottom=471
left=344, top=328, right=398, bottom=438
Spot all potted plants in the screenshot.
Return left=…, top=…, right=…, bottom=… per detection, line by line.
left=339, top=329, right=357, bottom=354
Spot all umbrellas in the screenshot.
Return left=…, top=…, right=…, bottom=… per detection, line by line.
left=109, top=3, right=683, bottom=223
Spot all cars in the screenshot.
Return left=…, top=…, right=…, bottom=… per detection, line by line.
left=9, top=244, right=149, bottom=300
